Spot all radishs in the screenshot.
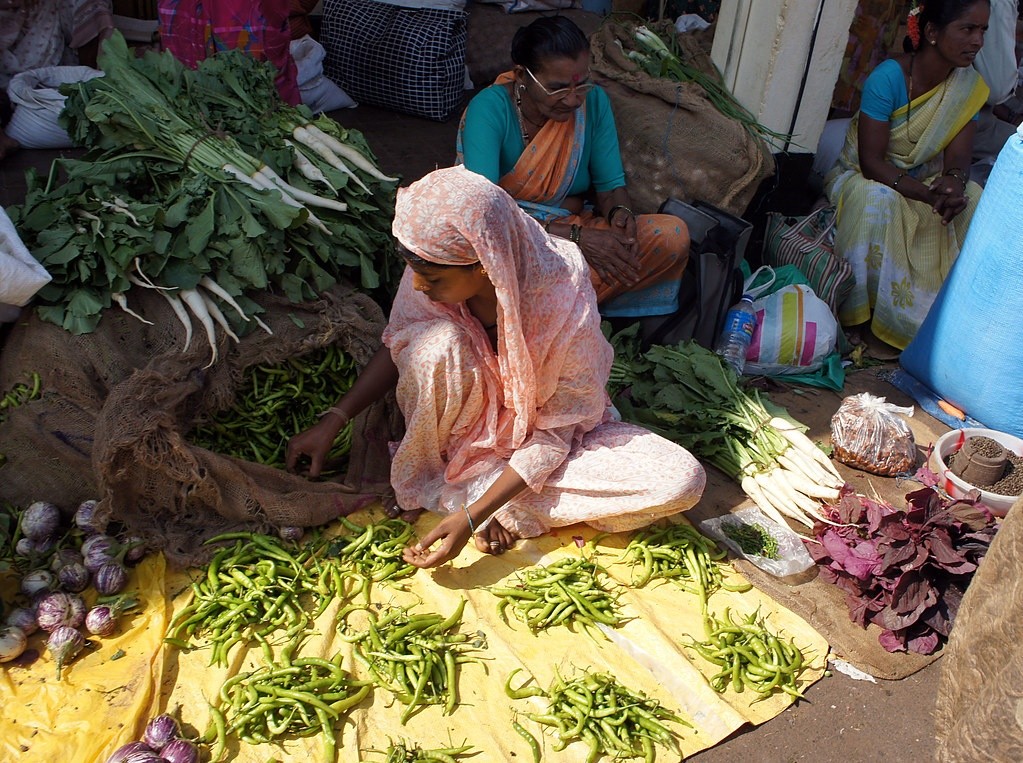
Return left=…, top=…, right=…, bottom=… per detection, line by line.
left=281, top=123, right=400, bottom=197
left=73, top=195, right=273, bottom=370
left=740, top=415, right=865, bottom=544
left=221, top=164, right=347, bottom=237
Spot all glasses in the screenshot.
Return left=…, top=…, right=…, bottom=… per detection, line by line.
left=521, top=64, right=594, bottom=100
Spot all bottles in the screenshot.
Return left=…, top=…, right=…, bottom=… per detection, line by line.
left=715, top=295, right=757, bottom=377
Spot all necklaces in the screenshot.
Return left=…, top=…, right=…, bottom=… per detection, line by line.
left=906, top=54, right=946, bottom=144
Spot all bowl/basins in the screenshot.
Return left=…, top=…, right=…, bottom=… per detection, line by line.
left=934, top=427, right=1023, bottom=517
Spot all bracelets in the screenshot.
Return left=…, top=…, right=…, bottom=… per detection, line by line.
left=892, top=174, right=903, bottom=189
left=543, top=221, right=551, bottom=230
left=570, top=224, right=583, bottom=245
left=461, top=504, right=475, bottom=538
left=607, top=205, right=632, bottom=227
left=946, top=167, right=967, bottom=191
left=316, top=407, right=348, bottom=425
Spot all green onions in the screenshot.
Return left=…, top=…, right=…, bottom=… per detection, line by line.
left=620, top=22, right=810, bottom=162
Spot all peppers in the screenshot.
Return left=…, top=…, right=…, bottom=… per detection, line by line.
left=183, top=340, right=360, bottom=482
left=0, top=371, right=40, bottom=424
left=162, top=509, right=818, bottom=762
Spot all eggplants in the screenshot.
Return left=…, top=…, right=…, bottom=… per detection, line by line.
left=0, top=500, right=148, bottom=681
left=105, top=712, right=203, bottom=763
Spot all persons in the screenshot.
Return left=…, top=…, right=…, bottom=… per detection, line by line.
left=285, top=165, right=706, bottom=569
left=958, top=0, right=1023, bottom=190
left=0, top=0, right=113, bottom=160
left=454, top=15, right=691, bottom=317
left=822, top=0, right=991, bottom=350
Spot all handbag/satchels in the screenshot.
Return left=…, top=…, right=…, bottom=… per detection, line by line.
left=320, top=2, right=470, bottom=124
left=762, top=206, right=853, bottom=330
left=829, top=392, right=918, bottom=477
left=737, top=256, right=846, bottom=389
left=157, top=0, right=302, bottom=110
